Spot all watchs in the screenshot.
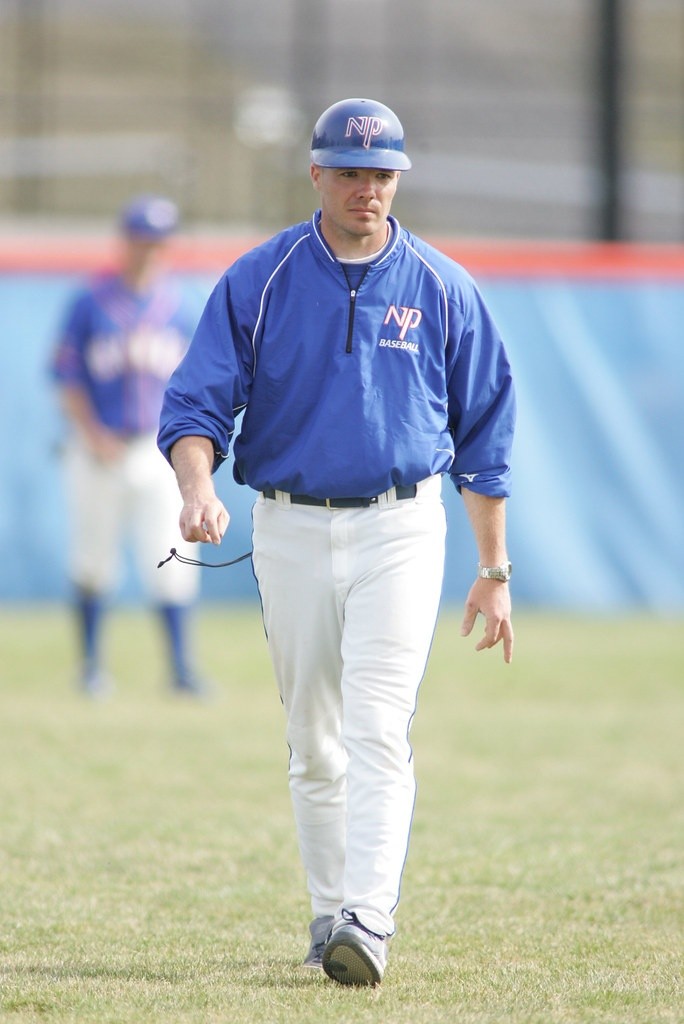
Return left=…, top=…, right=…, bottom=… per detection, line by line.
left=477, top=561, right=513, bottom=582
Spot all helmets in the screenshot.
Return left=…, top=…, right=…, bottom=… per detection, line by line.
left=311, top=98, right=412, bottom=172
left=121, top=195, right=179, bottom=245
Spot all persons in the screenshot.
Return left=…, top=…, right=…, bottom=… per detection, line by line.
left=45, top=197, right=211, bottom=695
left=155, top=98, right=516, bottom=987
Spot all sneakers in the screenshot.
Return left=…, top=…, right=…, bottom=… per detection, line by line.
left=303, top=916, right=335, bottom=971
left=322, top=908, right=395, bottom=987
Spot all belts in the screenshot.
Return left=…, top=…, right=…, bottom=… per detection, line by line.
left=261, top=483, right=417, bottom=511
